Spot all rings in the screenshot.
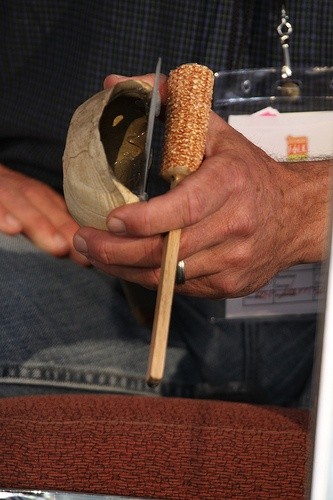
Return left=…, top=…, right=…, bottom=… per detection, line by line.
left=175, top=260, right=185, bottom=286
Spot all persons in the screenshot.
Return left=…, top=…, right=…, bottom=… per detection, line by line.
left=0, top=0, right=333, bottom=410
